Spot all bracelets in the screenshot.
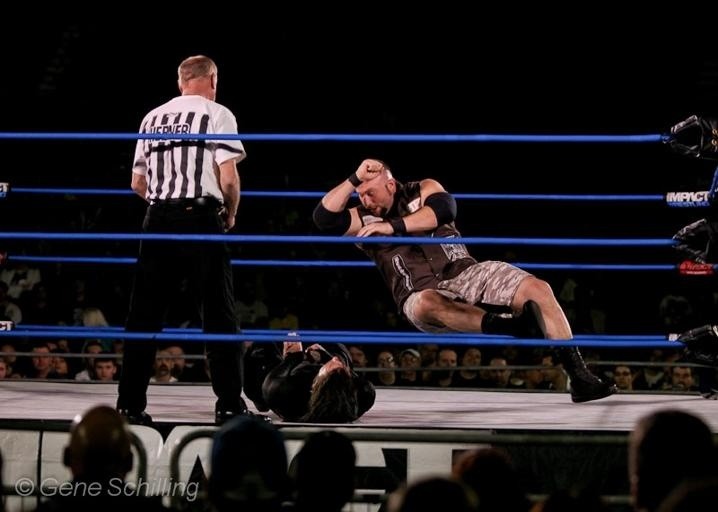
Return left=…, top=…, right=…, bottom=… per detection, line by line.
left=389, top=217, right=407, bottom=236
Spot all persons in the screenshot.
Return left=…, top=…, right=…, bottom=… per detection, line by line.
left=241, top=335, right=375, bottom=424
left=314, top=157, right=618, bottom=403
left=33, top=407, right=717, bottom=511
left=113, top=56, right=258, bottom=426
left=1, top=246, right=716, bottom=391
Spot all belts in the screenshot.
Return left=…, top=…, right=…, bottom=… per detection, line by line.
left=149, top=196, right=221, bottom=209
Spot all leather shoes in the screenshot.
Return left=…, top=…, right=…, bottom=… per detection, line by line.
left=114, top=408, right=151, bottom=423
left=215, top=409, right=271, bottom=423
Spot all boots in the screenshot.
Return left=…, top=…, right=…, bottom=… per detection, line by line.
left=552, top=344, right=617, bottom=403
left=481, top=300, right=547, bottom=339
